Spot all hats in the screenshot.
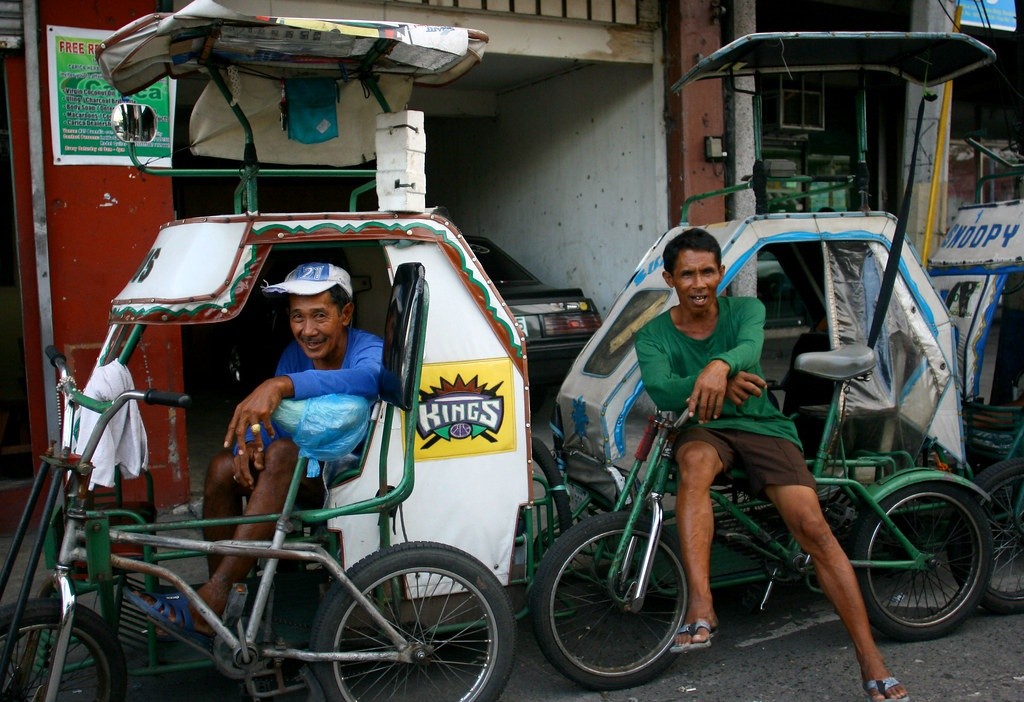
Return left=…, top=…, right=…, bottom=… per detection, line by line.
left=262, top=263, right=353, bottom=303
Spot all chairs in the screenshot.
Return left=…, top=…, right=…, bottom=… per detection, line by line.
left=729, top=333, right=833, bottom=502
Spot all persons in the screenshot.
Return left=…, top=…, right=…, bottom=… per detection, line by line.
left=633, top=228, right=912, bottom=702
left=122, top=262, right=384, bottom=647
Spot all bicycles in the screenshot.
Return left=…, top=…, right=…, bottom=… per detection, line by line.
left=0, top=262, right=533, bottom=702
left=529, top=342, right=998, bottom=692
left=944, top=410, right=1024, bottom=612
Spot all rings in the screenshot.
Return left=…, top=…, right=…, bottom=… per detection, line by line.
left=250, top=424, right=260, bottom=435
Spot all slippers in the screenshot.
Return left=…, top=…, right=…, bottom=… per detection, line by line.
left=863, top=676, right=910, bottom=702
left=669, top=621, right=720, bottom=653
left=121, top=587, right=211, bottom=648
